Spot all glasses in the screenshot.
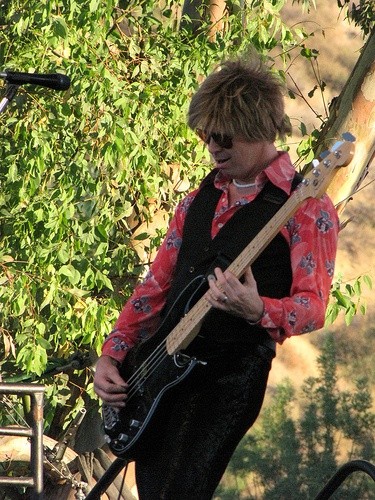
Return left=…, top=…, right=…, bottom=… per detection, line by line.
left=194, top=125, right=236, bottom=150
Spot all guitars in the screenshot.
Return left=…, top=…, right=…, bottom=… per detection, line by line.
left=100, top=131, right=357, bottom=458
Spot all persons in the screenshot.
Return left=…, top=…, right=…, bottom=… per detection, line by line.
left=94, top=54, right=339, bottom=500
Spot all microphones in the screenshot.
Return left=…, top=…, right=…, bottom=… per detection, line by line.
left=41, top=350, right=97, bottom=378
left=0, top=71, right=70, bottom=91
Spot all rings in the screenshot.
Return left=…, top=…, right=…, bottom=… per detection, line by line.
left=223, top=296, right=227, bottom=303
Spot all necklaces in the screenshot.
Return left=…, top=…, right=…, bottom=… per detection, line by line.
left=232, top=179, right=255, bottom=188
